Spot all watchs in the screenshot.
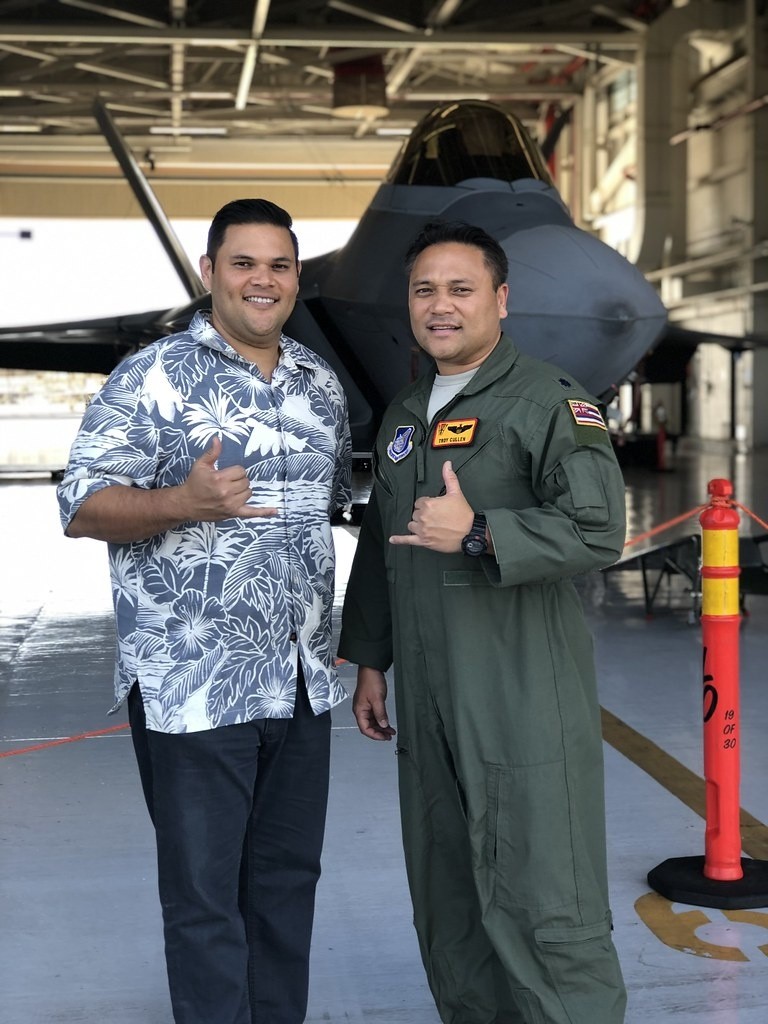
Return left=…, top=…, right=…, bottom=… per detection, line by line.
left=462, top=514, right=489, bottom=558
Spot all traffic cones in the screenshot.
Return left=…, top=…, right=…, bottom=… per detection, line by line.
left=641, top=480, right=767, bottom=914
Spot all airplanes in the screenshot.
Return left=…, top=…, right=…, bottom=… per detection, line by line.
left=0, top=97, right=767, bottom=445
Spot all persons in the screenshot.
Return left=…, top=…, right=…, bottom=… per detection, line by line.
left=57, top=198, right=351, bottom=1024
left=337, top=226, right=624, bottom=1024
